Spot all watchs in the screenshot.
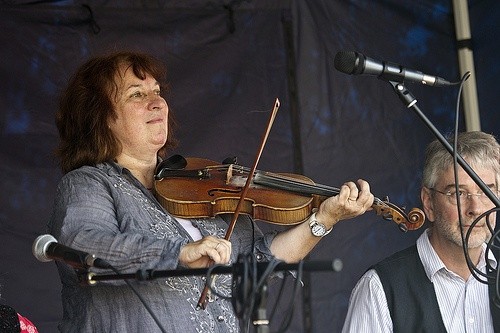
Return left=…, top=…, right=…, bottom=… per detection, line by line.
left=308, top=209, right=334, bottom=238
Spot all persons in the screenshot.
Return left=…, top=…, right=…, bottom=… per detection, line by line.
left=49, top=49, right=375, bottom=333
left=340, top=131, right=500, bottom=333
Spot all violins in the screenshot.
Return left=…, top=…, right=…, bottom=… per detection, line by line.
left=154, top=157, right=425, bottom=232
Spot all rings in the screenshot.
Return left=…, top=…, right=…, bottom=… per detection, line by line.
left=348, top=196, right=357, bottom=202
left=215, top=243, right=221, bottom=250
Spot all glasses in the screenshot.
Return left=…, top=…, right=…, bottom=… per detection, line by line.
left=423, top=184, right=500, bottom=206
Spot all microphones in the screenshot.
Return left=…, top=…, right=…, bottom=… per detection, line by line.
left=334, top=49, right=450, bottom=87
left=32, top=234, right=110, bottom=269
